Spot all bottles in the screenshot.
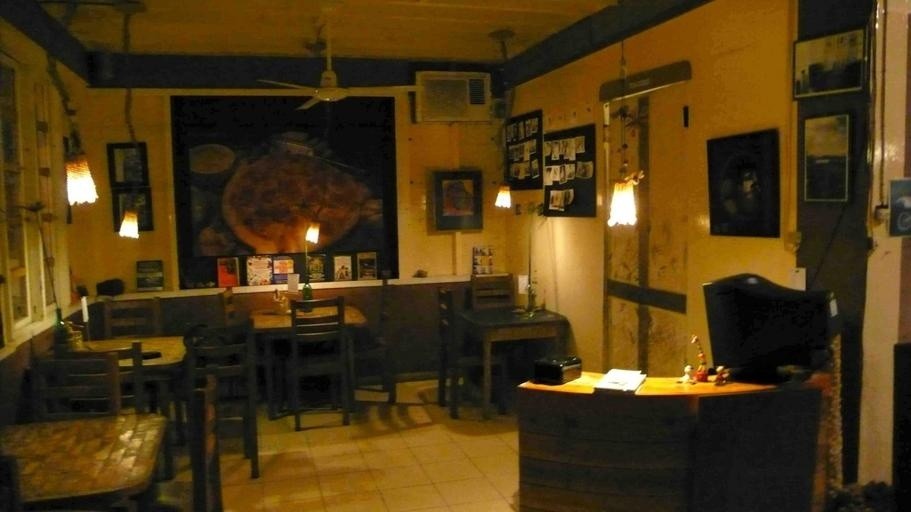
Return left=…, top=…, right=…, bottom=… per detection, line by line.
left=302, top=274, right=313, bottom=312
left=54, top=307, right=69, bottom=340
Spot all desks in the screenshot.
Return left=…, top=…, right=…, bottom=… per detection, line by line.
left=252, top=305, right=368, bottom=420
left=461, top=308, right=571, bottom=418
left=515, top=373, right=832, bottom=510
left=52, top=335, right=187, bottom=450
left=4, top=410, right=165, bottom=512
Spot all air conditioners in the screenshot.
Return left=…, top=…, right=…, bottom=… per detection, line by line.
left=409, top=70, right=494, bottom=126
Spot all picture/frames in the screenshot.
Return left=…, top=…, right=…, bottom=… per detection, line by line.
left=789, top=26, right=865, bottom=97
left=432, top=167, right=485, bottom=232
left=705, top=126, right=781, bottom=239
left=106, top=140, right=156, bottom=235
left=799, top=110, right=856, bottom=211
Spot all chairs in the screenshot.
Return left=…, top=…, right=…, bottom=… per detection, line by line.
left=103, top=294, right=163, bottom=335
left=149, top=373, right=223, bottom=511
left=277, top=295, right=353, bottom=431
left=29, top=348, right=122, bottom=416
left=149, top=319, right=261, bottom=481
left=433, top=274, right=514, bottom=420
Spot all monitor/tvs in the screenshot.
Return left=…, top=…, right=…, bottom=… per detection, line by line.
left=702, top=271, right=833, bottom=385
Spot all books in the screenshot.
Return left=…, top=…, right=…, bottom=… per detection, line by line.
left=246, top=254, right=274, bottom=286
left=216, top=256, right=242, bottom=287
left=272, top=255, right=296, bottom=284
left=357, top=251, right=378, bottom=280
left=136, top=260, right=167, bottom=291
left=332, top=254, right=354, bottom=281
left=594, top=368, right=650, bottom=397
left=306, top=253, right=329, bottom=282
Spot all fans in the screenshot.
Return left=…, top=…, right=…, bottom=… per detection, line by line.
left=256, top=26, right=422, bottom=110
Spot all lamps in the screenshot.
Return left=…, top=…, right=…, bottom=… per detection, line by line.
left=44, top=1, right=101, bottom=208
left=606, top=36, right=645, bottom=227
left=108, top=1, right=147, bottom=246
left=487, top=28, right=519, bottom=212
left=303, top=155, right=329, bottom=245
